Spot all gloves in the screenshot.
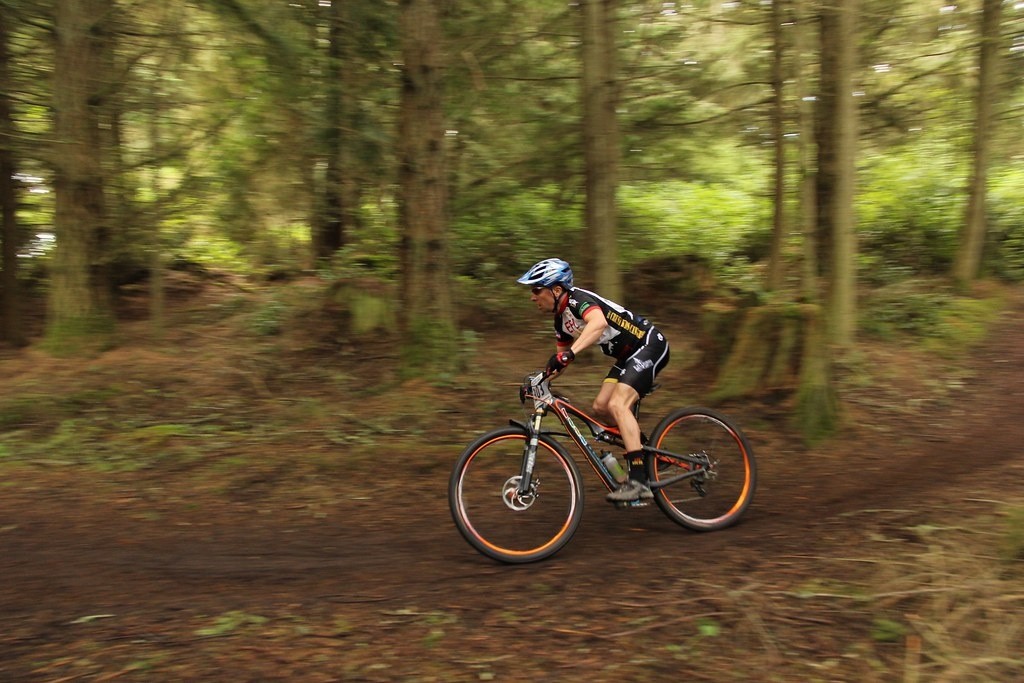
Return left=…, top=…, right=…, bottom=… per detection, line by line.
left=520, top=384, right=534, bottom=404
left=546, top=349, right=575, bottom=377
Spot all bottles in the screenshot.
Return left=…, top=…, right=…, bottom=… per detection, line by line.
left=599, top=450, right=627, bottom=483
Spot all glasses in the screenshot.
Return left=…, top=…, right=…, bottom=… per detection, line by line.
left=532, top=285, right=547, bottom=295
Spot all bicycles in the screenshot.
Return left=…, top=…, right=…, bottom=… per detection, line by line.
left=447, top=356, right=760, bottom=565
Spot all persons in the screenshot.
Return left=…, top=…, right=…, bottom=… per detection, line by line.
left=516, top=258, right=671, bottom=502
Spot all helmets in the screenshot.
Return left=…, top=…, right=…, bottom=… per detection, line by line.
left=516, top=257, right=574, bottom=289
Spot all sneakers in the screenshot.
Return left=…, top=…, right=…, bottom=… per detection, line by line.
left=654, top=456, right=671, bottom=471
left=605, top=478, right=656, bottom=503
